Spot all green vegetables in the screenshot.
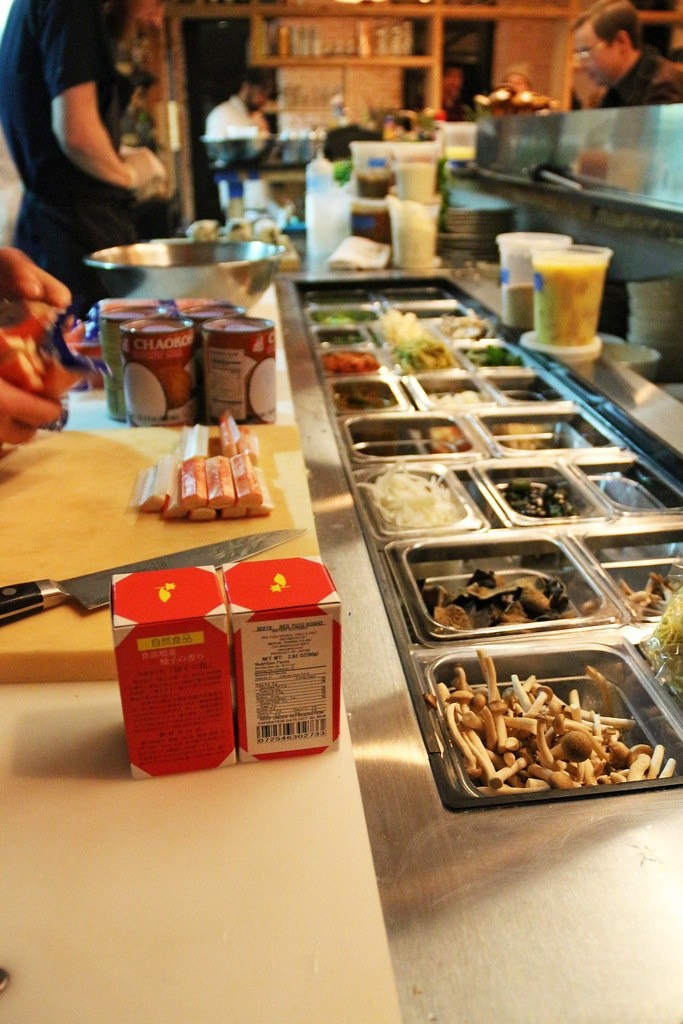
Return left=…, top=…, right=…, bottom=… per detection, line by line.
left=503, top=480, right=580, bottom=516
left=319, top=315, right=376, bottom=345
left=465, top=346, right=525, bottom=367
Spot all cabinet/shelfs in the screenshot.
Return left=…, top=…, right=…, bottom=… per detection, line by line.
left=247, top=4, right=443, bottom=113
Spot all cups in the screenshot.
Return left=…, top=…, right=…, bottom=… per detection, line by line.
left=495, top=232, right=575, bottom=332
left=305, top=140, right=444, bottom=271
left=531, top=244, right=613, bottom=347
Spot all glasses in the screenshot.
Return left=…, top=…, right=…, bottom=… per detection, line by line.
left=573, top=42, right=606, bottom=63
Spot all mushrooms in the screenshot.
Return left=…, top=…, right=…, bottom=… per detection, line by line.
left=422, top=650, right=677, bottom=796
left=617, top=571, right=678, bottom=617
left=417, top=568, right=575, bottom=630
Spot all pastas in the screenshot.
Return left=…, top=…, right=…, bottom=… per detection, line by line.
left=640, top=587, right=683, bottom=703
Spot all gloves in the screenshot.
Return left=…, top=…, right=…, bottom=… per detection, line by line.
left=119, top=145, right=167, bottom=202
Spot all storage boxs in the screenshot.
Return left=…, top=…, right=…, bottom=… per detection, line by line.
left=109, top=565, right=236, bottom=781
left=221, top=557, right=343, bottom=762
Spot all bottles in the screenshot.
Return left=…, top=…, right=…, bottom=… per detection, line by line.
left=265, top=23, right=414, bottom=59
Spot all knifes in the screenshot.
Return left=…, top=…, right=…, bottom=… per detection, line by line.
left=0, top=527, right=313, bottom=628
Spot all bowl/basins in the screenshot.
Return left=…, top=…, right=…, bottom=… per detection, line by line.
left=82, top=236, right=287, bottom=309
left=600, top=271, right=683, bottom=382
left=200, top=135, right=326, bottom=164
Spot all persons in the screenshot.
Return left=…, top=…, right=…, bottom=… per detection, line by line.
left=0, top=0, right=169, bottom=300
left=204, top=69, right=270, bottom=138
left=0, top=248, right=83, bottom=444
left=569, top=0, right=683, bottom=110
left=502, top=62, right=534, bottom=91
left=441, top=61, right=473, bottom=123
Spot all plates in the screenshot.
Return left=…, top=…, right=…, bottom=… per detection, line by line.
left=439, top=205, right=520, bottom=251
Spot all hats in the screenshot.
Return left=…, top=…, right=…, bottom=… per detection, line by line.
left=504, top=62, right=536, bottom=89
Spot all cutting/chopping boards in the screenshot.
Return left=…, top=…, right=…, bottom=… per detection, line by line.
left=0, top=427, right=322, bottom=683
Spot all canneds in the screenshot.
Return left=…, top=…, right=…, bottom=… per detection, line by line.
left=96, top=302, right=276, bottom=427
